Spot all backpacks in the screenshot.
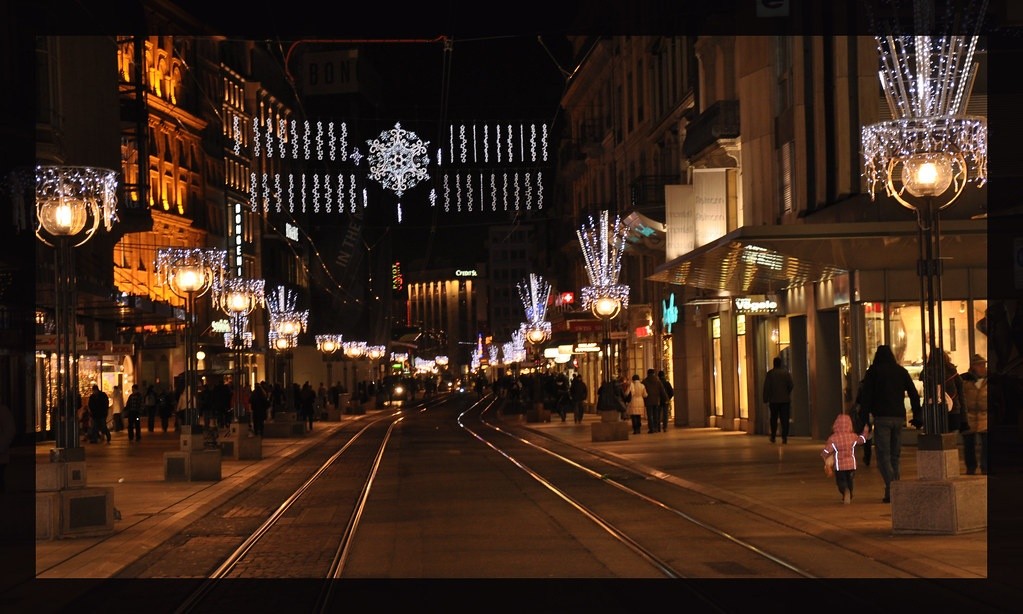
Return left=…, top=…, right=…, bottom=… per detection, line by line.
left=145, top=395, right=155, bottom=406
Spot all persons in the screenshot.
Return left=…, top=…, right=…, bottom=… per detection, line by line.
left=959, top=354, right=988, bottom=473
left=490, top=370, right=588, bottom=423
left=854, top=345, right=923, bottom=502
left=821, top=413, right=869, bottom=505
left=597, top=369, right=674, bottom=434
left=763, top=358, right=794, bottom=444
left=79, top=374, right=439, bottom=444
left=919, top=349, right=971, bottom=435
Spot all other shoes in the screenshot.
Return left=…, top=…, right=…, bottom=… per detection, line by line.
left=843, top=490, right=851, bottom=505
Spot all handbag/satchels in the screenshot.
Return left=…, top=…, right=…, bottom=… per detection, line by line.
left=624, top=391, right=632, bottom=403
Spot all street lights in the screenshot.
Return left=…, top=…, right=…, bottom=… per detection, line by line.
left=319, top=339, right=339, bottom=407
left=169, top=255, right=215, bottom=436
left=219, top=288, right=256, bottom=424
left=345, top=345, right=363, bottom=401
left=526, top=324, right=548, bottom=404
left=885, top=132, right=970, bottom=451
left=368, top=348, right=381, bottom=394
left=34, top=190, right=100, bottom=463
left=591, top=291, right=622, bottom=410
left=275, top=319, right=303, bottom=413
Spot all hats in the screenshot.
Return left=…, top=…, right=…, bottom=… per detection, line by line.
left=971, top=354, right=987, bottom=364
